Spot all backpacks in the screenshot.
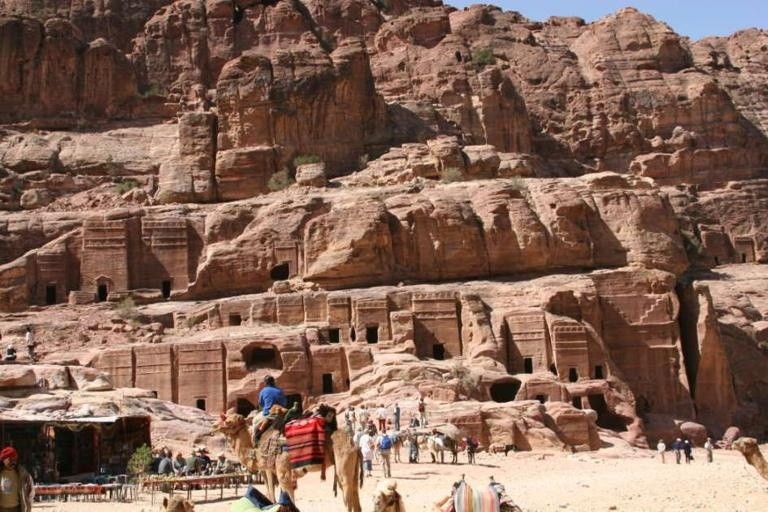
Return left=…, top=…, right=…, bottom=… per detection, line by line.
left=379, top=434, right=391, bottom=449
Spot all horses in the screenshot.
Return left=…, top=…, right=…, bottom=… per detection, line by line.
left=373, top=428, right=413, bottom=464
left=421, top=432, right=459, bottom=465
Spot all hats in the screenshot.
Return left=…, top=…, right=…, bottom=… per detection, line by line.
left=0, top=446, right=18, bottom=462
left=264, top=375, right=275, bottom=384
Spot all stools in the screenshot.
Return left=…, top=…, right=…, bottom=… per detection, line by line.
left=33, top=483, right=131, bottom=503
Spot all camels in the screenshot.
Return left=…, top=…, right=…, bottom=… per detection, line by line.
left=374, top=473, right=521, bottom=512
left=731, top=436, right=768, bottom=482
left=208, top=402, right=364, bottom=512
left=162, top=495, right=195, bottom=512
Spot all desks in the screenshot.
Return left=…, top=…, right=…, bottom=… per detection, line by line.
left=151, top=473, right=249, bottom=505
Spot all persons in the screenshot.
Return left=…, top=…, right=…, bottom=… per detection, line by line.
left=672, top=437, right=683, bottom=464
left=250, top=373, right=289, bottom=459
left=0, top=446, right=37, bottom=512
left=657, top=439, right=667, bottom=463
left=149, top=444, right=235, bottom=490
left=24, top=327, right=36, bottom=362
left=682, top=439, right=692, bottom=464
left=371, top=478, right=407, bottom=511
left=704, top=437, right=714, bottom=462
left=343, top=398, right=478, bottom=478
left=4, top=344, right=16, bottom=360
